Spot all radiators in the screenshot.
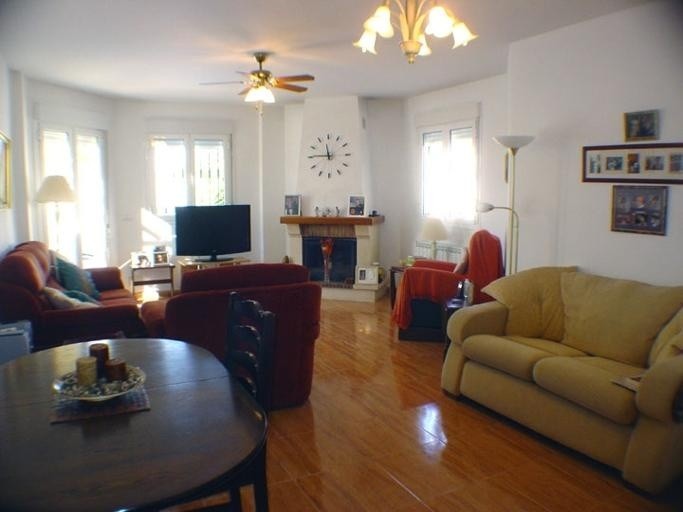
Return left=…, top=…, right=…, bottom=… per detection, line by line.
left=411, top=238, right=461, bottom=265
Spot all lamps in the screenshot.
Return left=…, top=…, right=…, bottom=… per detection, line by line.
left=416, top=218, right=449, bottom=261
left=29, top=174, right=77, bottom=224
left=473, top=200, right=522, bottom=274
left=241, top=85, right=277, bottom=119
left=349, top=1, right=478, bottom=69
left=488, top=132, right=538, bottom=274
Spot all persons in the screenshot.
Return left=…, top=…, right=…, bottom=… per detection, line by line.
left=642, top=117, right=654, bottom=135
left=627, top=116, right=641, bottom=137
left=628, top=188, right=650, bottom=228
left=155, top=254, right=164, bottom=263
left=136, top=256, right=150, bottom=266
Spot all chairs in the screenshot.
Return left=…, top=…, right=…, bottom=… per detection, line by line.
left=391, top=226, right=507, bottom=348
left=138, top=261, right=324, bottom=414
left=179, top=284, right=281, bottom=511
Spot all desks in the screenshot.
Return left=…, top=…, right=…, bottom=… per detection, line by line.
left=388, top=265, right=410, bottom=311
left=434, top=298, right=479, bottom=362
left=127, top=261, right=176, bottom=307
left=0, top=330, right=270, bottom=512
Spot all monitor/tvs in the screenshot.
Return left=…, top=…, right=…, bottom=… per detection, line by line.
left=175, top=205, right=250, bottom=262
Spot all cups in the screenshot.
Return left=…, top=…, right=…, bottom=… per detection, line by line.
left=406, top=255, right=415, bottom=267
left=398, top=258, right=407, bottom=266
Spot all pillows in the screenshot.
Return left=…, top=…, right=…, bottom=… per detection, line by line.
left=450, top=245, right=472, bottom=276
left=60, top=285, right=99, bottom=305
left=41, top=283, right=81, bottom=311
left=46, top=246, right=102, bottom=301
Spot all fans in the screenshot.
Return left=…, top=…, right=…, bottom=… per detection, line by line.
left=197, top=49, right=315, bottom=97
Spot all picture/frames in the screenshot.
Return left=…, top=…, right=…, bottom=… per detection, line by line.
left=620, top=107, right=662, bottom=143
left=347, top=194, right=367, bottom=217
left=0, top=130, right=12, bottom=212
left=609, top=184, right=669, bottom=238
left=580, top=141, right=681, bottom=187
left=282, top=192, right=301, bottom=217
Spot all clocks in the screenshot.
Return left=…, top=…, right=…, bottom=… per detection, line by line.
left=302, top=132, right=353, bottom=179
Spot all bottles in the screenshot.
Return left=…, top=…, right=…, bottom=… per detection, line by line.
left=468, top=281, right=473, bottom=302
left=76, top=341, right=128, bottom=385
left=455, top=280, right=463, bottom=299
left=463, top=276, right=470, bottom=302
left=462, top=296, right=471, bottom=310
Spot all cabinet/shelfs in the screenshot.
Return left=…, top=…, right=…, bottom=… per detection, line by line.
left=178, top=255, right=253, bottom=275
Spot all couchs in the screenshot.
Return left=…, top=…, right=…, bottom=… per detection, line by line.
left=429, top=261, right=682, bottom=501
left=0, top=239, right=148, bottom=351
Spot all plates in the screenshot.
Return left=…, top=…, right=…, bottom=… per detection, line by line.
left=49, top=365, right=146, bottom=405
